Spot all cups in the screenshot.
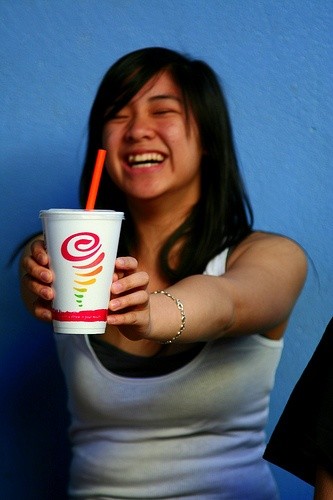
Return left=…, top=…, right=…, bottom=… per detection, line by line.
left=40, top=208, right=124, bottom=334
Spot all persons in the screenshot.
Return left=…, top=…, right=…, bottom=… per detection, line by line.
left=17, top=47, right=309, bottom=500
left=263, top=315, right=333, bottom=500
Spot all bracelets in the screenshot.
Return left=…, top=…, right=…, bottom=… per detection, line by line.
left=148, top=290, right=186, bottom=346
